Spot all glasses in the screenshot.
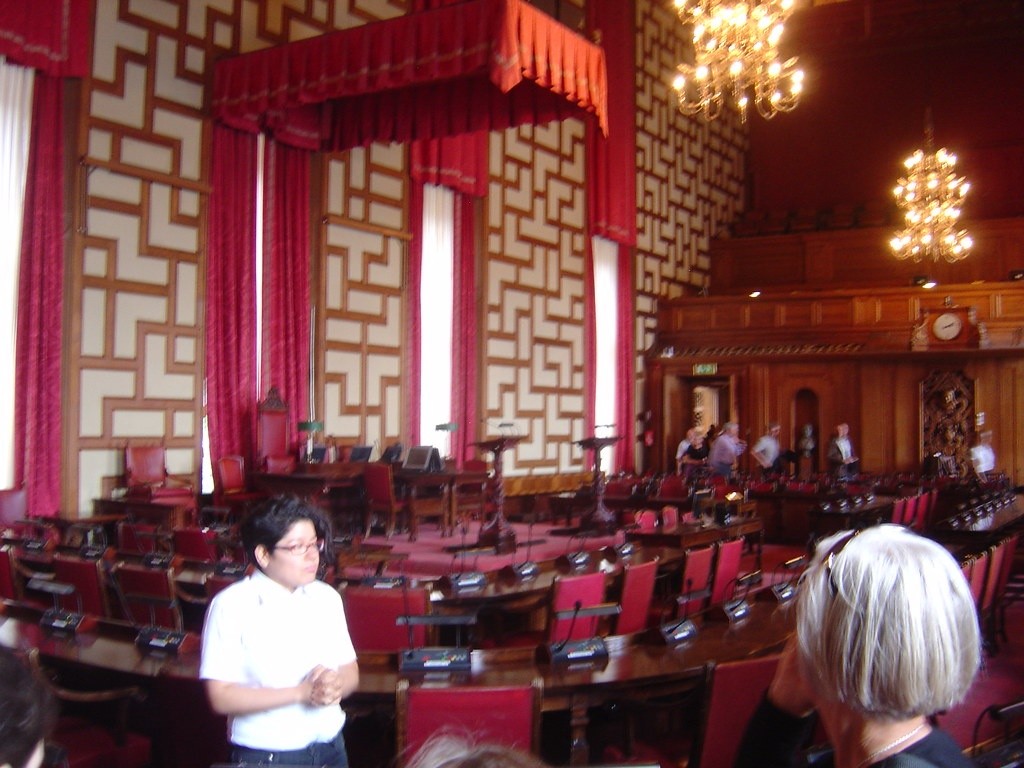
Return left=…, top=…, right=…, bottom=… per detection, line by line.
left=272, top=539, right=325, bottom=555
left=821, top=529, right=860, bottom=597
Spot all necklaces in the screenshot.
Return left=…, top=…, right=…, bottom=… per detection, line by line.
left=853, top=721, right=928, bottom=768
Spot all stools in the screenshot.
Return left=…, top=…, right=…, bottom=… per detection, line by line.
left=548, top=493, right=576, bottom=528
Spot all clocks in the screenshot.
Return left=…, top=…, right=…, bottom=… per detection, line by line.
left=932, top=313, right=963, bottom=341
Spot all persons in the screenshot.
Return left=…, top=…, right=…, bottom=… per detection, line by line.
left=199, top=496, right=359, bottom=768
left=733, top=523, right=984, bottom=768
left=675, top=422, right=859, bottom=481
left=969, top=429, right=995, bottom=488
left=0, top=644, right=69, bottom=768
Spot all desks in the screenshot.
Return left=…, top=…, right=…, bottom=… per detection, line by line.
left=0, top=577, right=805, bottom=768
left=0, top=537, right=689, bottom=648
left=265, top=472, right=359, bottom=508
left=929, top=494, right=1024, bottom=543
left=804, top=495, right=894, bottom=531
left=395, top=472, right=453, bottom=543
left=447, top=471, right=491, bottom=537
left=625, top=516, right=766, bottom=584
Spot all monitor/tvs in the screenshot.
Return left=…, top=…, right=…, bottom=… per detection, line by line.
left=302, top=443, right=444, bottom=475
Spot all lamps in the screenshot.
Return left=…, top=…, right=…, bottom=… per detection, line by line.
left=671, top=0, right=805, bottom=124
left=884, top=90, right=973, bottom=262
left=912, top=274, right=937, bottom=289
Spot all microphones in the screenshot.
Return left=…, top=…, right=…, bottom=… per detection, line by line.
left=0, top=518, right=802, bottom=673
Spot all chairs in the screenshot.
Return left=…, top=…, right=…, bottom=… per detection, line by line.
left=0, top=380, right=1024, bottom=768
